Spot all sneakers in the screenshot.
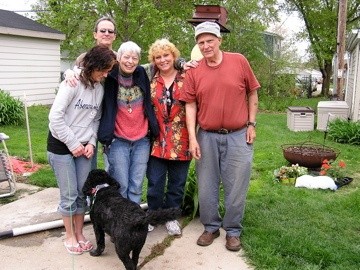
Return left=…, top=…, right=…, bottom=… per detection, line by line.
left=165, top=220, right=181, bottom=235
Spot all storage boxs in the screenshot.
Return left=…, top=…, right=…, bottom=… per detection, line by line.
left=318, top=100, right=349, bottom=130
left=286, top=107, right=315, bottom=131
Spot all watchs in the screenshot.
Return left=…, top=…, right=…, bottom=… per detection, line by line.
left=246, top=121, right=257, bottom=128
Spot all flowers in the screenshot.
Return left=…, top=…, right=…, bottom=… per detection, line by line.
left=273, top=163, right=308, bottom=177
left=321, top=160, right=345, bottom=178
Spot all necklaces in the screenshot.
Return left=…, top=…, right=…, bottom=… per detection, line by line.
left=118, top=74, right=133, bottom=114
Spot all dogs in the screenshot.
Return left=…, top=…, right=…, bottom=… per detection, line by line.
left=82, top=169, right=183, bottom=270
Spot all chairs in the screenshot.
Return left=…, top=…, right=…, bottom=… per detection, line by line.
left=0, top=133, right=17, bottom=199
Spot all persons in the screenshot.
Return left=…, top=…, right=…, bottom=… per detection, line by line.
left=179, top=21, right=261, bottom=251
left=46, top=43, right=120, bottom=255
left=63, top=41, right=199, bottom=206
left=146, top=38, right=248, bottom=236
left=70, top=17, right=117, bottom=69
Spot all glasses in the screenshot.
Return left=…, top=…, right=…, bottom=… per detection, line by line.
left=97, top=29, right=115, bottom=33
left=164, top=90, right=171, bottom=106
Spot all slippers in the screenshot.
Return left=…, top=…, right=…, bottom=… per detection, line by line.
left=64, top=241, right=83, bottom=254
left=78, top=240, right=93, bottom=252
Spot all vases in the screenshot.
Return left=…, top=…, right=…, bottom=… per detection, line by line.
left=282, top=178, right=295, bottom=186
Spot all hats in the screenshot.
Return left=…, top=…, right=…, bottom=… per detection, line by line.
left=194, top=22, right=222, bottom=43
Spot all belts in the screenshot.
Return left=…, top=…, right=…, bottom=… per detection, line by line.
left=200, top=123, right=247, bottom=134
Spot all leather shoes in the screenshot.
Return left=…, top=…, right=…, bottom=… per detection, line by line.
left=226, top=234, right=241, bottom=252
left=197, top=229, right=220, bottom=247
